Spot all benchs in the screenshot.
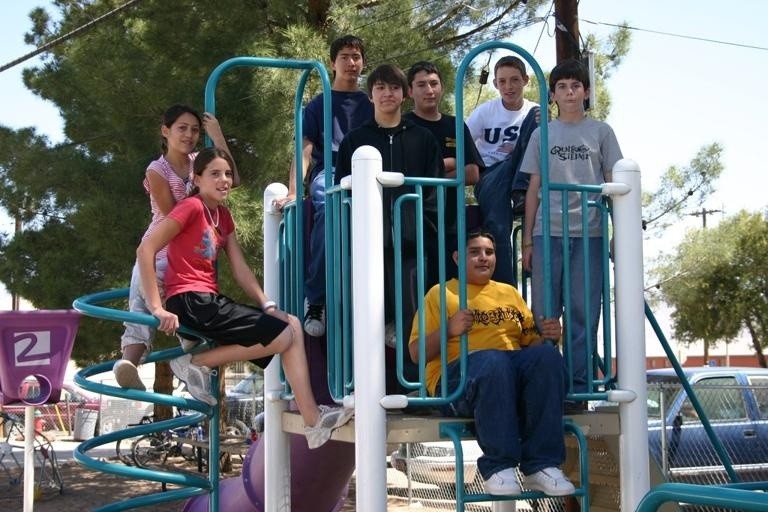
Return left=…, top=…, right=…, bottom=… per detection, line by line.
left=172, top=433, right=253, bottom=474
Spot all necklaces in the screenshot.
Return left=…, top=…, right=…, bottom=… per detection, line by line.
left=201, top=200, right=220, bottom=227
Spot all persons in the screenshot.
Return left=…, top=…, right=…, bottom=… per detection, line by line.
left=271, top=35, right=396, bottom=349
left=408, top=231, right=575, bottom=497
left=335, top=64, right=446, bottom=416
left=136, top=147, right=355, bottom=450
left=402, top=61, right=486, bottom=295
left=465, top=56, right=552, bottom=289
left=518, top=59, right=624, bottom=409
left=113, top=105, right=241, bottom=392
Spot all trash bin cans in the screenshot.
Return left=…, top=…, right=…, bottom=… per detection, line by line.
left=74, top=408, right=98, bottom=442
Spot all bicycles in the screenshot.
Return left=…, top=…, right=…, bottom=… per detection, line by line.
left=115, top=405, right=205, bottom=470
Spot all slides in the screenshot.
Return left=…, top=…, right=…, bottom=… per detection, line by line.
left=182, top=395, right=355, bottom=512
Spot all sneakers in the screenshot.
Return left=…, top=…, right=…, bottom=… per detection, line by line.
left=522, top=467, right=575, bottom=497
left=175, top=332, right=199, bottom=354
left=169, top=353, right=218, bottom=407
left=113, top=359, right=147, bottom=392
left=483, top=467, right=521, bottom=497
left=565, top=398, right=583, bottom=413
left=301, top=405, right=355, bottom=450
left=404, top=390, right=433, bottom=415
left=510, top=189, right=527, bottom=216
left=303, top=295, right=326, bottom=337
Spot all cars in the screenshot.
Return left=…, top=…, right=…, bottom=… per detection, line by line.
left=391, top=440, right=485, bottom=499
left=589, top=366, right=768, bottom=474
left=1, top=384, right=122, bottom=440
left=224, top=374, right=264, bottom=433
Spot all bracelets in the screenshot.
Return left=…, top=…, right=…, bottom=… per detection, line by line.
left=260, top=301, right=277, bottom=311
left=522, top=243, right=532, bottom=250
